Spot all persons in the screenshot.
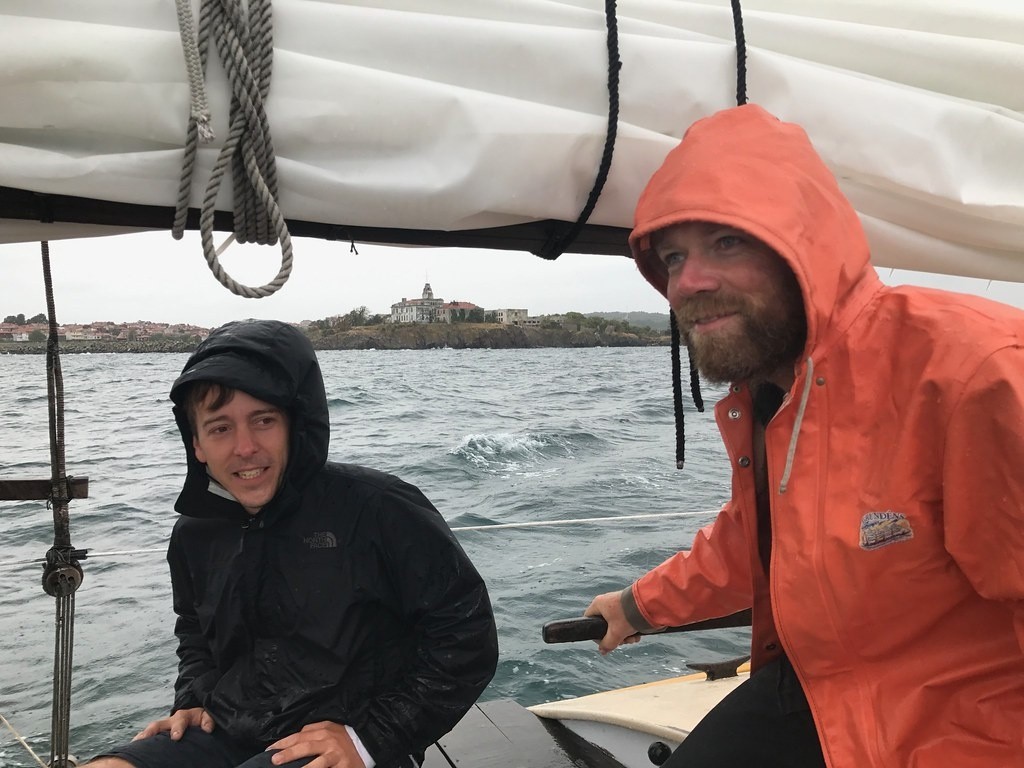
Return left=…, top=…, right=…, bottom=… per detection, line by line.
left=585, top=103, right=1022, bottom=767
left=77, top=321, right=499, bottom=768
